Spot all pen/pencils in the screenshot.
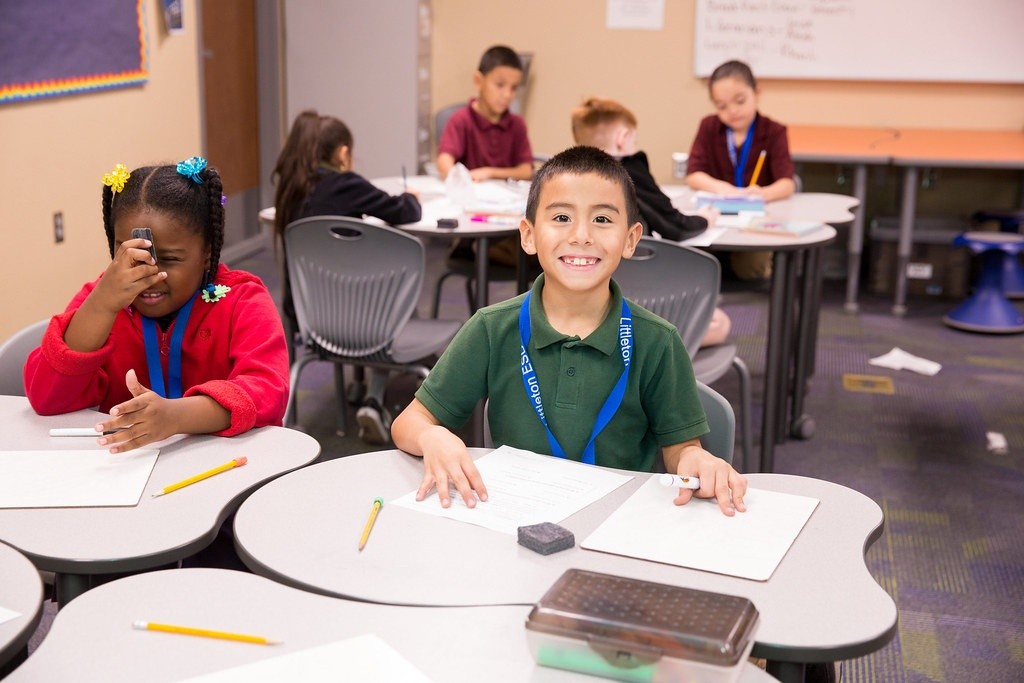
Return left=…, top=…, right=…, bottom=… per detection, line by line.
left=659, top=474, right=700, bottom=491
left=150, top=456, right=250, bottom=498
left=130, top=620, right=285, bottom=646
left=48, top=427, right=127, bottom=437
left=402, top=166, right=407, bottom=191
left=749, top=149, right=767, bottom=187
left=718, top=211, right=752, bottom=216
left=357, top=496, right=383, bottom=550
left=471, top=216, right=518, bottom=226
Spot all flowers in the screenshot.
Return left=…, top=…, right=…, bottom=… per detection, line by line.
left=257, top=176, right=533, bottom=405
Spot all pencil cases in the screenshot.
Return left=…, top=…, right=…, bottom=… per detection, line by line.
left=521, top=567, right=760, bottom=682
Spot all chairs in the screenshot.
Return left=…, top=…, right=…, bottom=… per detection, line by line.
left=281, top=215, right=462, bottom=427
left=611, top=236, right=756, bottom=474
left=430, top=257, right=518, bottom=320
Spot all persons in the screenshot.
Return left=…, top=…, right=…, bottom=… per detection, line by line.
left=686, top=60, right=796, bottom=289
left=436, top=45, right=533, bottom=270
left=24, top=156, right=290, bottom=454
left=391, top=145, right=748, bottom=517
left=571, top=97, right=731, bottom=347
left=271, top=110, right=422, bottom=446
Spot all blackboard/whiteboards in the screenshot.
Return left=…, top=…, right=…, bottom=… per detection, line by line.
left=693, top=0, right=1024, bottom=84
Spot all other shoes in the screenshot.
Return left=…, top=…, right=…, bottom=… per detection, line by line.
left=356, top=397, right=390, bottom=444
left=345, top=383, right=364, bottom=404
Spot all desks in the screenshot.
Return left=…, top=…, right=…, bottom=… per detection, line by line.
left=0, top=541, right=45, bottom=679
left=654, top=188, right=861, bottom=473
left=893, top=128, right=1024, bottom=316
left=0, top=567, right=783, bottom=683
left=0, top=394, right=322, bottom=612
left=780, top=124, right=894, bottom=311
left=231, top=447, right=899, bottom=683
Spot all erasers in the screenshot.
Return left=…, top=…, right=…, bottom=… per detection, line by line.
left=437, top=218, right=457, bottom=228
left=518, top=522, right=576, bottom=555
